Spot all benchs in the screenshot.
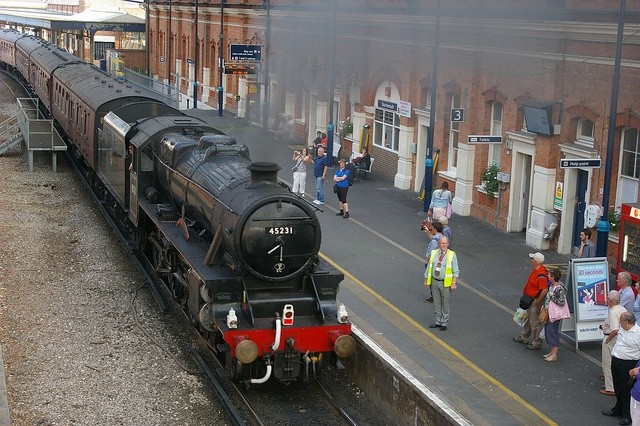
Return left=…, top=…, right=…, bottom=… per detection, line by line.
left=308, top=140, right=342, bottom=159
left=338, top=150, right=375, bottom=181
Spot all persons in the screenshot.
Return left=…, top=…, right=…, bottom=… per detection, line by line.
left=332, top=159, right=350, bottom=218
left=351, top=147, right=370, bottom=170
left=576, top=229, right=597, bottom=258
left=542, top=268, right=565, bottom=364
left=633, top=280, right=640, bottom=327
left=311, top=130, right=328, bottom=154
left=290, top=146, right=310, bottom=196
left=512, top=251, right=549, bottom=351
left=427, top=180, right=453, bottom=221
left=597, top=290, right=630, bottom=395
left=438, top=215, right=454, bottom=251
left=604, top=310, right=638, bottom=425
left=425, top=234, right=460, bottom=330
left=614, top=270, right=635, bottom=319
left=307, top=147, right=328, bottom=203
left=422, top=222, right=444, bottom=302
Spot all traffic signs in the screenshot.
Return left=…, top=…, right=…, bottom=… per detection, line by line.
left=231, top=44, right=261, bottom=62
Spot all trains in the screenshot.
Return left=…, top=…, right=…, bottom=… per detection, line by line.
left=0, top=28, right=357, bottom=391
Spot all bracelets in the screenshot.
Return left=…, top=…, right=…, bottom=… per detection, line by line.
left=323, top=173, right=326, bottom=175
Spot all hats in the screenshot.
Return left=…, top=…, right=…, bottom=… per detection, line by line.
left=529, top=252, right=544, bottom=264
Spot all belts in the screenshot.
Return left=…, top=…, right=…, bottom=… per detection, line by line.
left=604, top=333, right=609, bottom=335
left=432, top=276, right=444, bottom=282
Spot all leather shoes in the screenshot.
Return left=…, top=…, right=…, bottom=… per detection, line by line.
left=599, top=389, right=616, bottom=395
left=620, top=418, right=631, bottom=426
left=602, top=409, right=615, bottom=416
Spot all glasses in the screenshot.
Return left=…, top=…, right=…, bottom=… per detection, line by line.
left=606, top=297, right=610, bottom=300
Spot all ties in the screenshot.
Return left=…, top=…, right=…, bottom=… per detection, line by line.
left=435, top=253, right=444, bottom=276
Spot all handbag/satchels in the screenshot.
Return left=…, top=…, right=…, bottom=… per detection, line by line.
left=292, top=166, right=298, bottom=172
left=538, top=308, right=548, bottom=324
left=519, top=294, right=533, bottom=310
left=549, top=284, right=572, bottom=322
left=421, top=219, right=433, bottom=231
left=333, top=184, right=338, bottom=193
left=446, top=203, right=452, bottom=218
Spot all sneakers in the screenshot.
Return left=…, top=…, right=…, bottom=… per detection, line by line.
left=542, top=353, right=552, bottom=357
left=336, top=212, right=343, bottom=216
left=513, top=336, right=528, bottom=344
left=343, top=213, right=349, bottom=218
left=313, top=199, right=318, bottom=203
left=544, top=356, right=557, bottom=362
left=440, top=326, right=447, bottom=331
left=316, top=201, right=324, bottom=205
left=424, top=297, right=434, bottom=302
left=527, top=343, right=536, bottom=349
left=302, top=194, right=305, bottom=198
left=429, top=323, right=441, bottom=328
